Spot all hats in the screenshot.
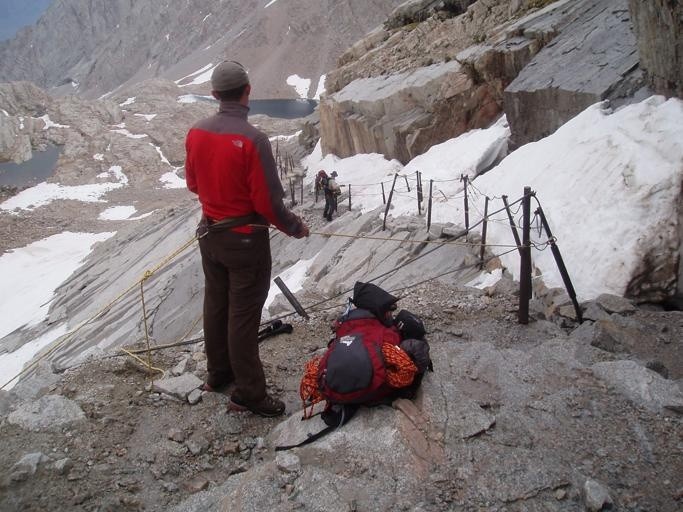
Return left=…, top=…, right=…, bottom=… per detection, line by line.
left=211, top=59, right=250, bottom=92
left=331, top=171, right=338, bottom=177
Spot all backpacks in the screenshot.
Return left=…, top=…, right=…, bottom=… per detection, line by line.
left=319, top=308, right=403, bottom=408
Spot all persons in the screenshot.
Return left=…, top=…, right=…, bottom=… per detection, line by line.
left=323, top=170, right=341, bottom=222
left=186, top=59, right=311, bottom=418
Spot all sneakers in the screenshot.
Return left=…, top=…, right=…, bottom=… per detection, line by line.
left=229, top=395, right=286, bottom=417
left=203, top=375, right=235, bottom=392
